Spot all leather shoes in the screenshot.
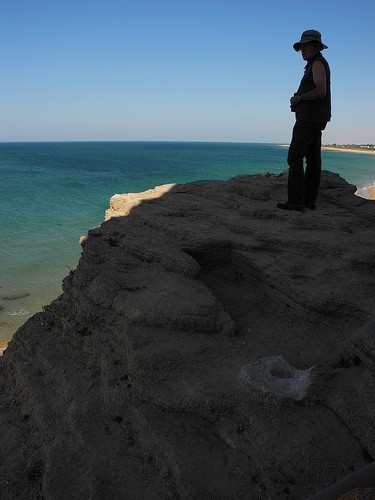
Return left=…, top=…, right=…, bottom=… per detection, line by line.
left=277, top=203, right=304, bottom=211
left=304, top=202, right=315, bottom=209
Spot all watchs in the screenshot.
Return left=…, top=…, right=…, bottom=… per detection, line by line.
left=296, top=92, right=303, bottom=103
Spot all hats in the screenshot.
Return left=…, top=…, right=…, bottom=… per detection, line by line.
left=293, top=30, right=329, bottom=52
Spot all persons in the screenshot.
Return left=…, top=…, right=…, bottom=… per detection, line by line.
left=275, top=28, right=333, bottom=213
